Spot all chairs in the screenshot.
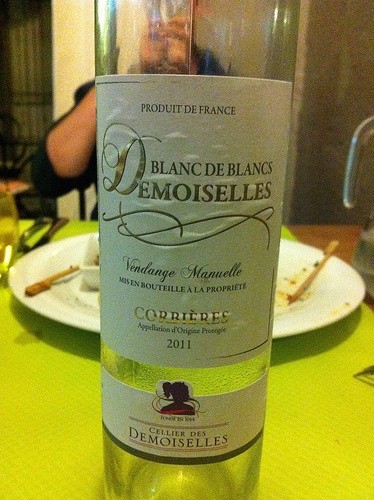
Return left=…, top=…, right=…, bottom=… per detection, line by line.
left=1, top=111, right=22, bottom=162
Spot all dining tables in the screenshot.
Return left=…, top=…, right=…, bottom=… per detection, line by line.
left=1, top=221, right=373, bottom=500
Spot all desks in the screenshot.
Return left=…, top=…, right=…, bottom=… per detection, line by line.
left=1, top=138, right=43, bottom=218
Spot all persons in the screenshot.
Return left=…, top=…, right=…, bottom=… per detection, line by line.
left=31, top=0, right=237, bottom=221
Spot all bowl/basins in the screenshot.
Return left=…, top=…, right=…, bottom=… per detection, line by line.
left=80, top=236, right=100, bottom=288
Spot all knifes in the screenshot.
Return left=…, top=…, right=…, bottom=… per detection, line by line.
left=31, top=218, right=69, bottom=250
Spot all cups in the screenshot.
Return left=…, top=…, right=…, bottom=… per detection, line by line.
left=0, top=188, right=19, bottom=285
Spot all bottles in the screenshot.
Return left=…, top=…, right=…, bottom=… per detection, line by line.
left=93, top=0, right=302, bottom=500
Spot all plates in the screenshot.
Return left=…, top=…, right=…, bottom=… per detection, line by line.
left=7, top=231, right=366, bottom=340
